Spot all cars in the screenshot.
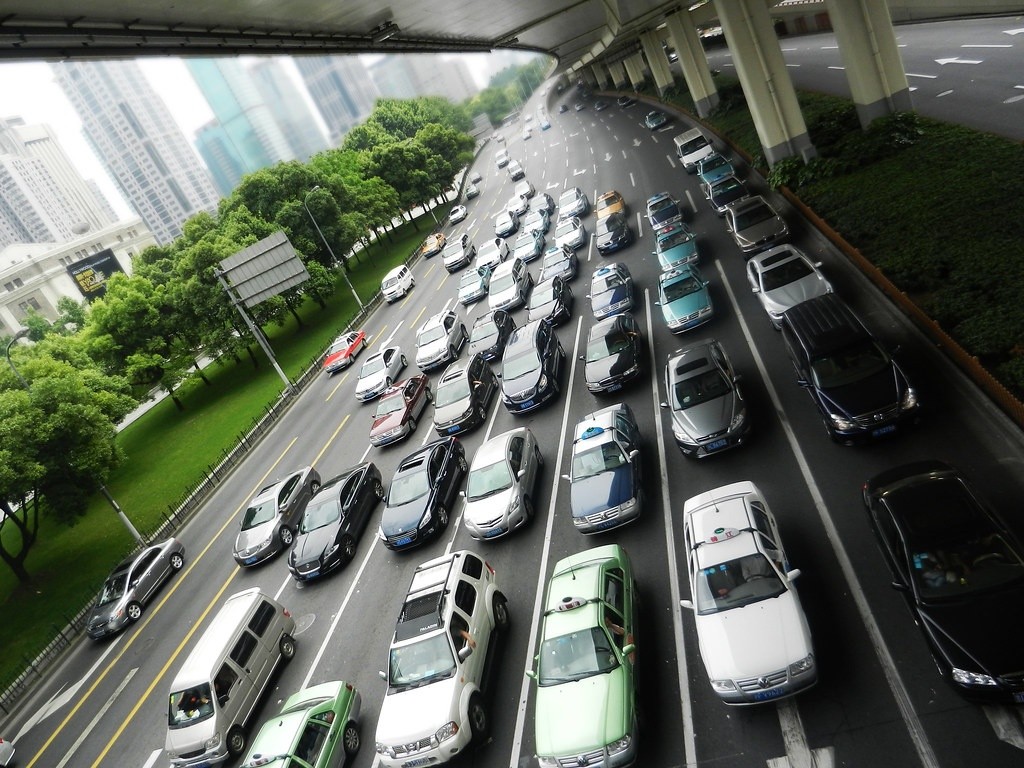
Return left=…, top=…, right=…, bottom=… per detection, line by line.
left=229, top=401, right=647, bottom=582
left=642, top=126, right=1024, bottom=706
left=523, top=83, right=551, bottom=131
left=323, top=331, right=368, bottom=374
left=85, top=536, right=185, bottom=641
left=420, top=233, right=447, bottom=258
left=449, top=172, right=482, bottom=224
left=355, top=186, right=642, bottom=449
left=667, top=52, right=678, bottom=64
left=559, top=91, right=632, bottom=114
left=239, top=680, right=362, bottom=768
left=523, top=543, right=638, bottom=768
left=491, top=132, right=536, bottom=198
left=0, top=737, right=16, bottom=768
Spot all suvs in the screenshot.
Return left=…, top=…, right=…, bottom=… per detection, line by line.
left=369, top=551, right=510, bottom=768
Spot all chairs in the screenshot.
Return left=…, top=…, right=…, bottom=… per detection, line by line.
left=582, top=453, right=600, bottom=470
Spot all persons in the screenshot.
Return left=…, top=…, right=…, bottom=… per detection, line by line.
left=201, top=682, right=224, bottom=704
left=612, top=331, right=641, bottom=346
left=740, top=548, right=782, bottom=582
left=433, top=627, right=476, bottom=659
left=588, top=617, right=625, bottom=652
left=605, top=441, right=629, bottom=460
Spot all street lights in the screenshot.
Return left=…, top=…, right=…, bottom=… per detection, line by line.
left=6, top=325, right=149, bottom=550
left=304, top=186, right=367, bottom=315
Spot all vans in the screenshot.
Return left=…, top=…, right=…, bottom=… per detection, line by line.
left=380, top=265, right=415, bottom=305
left=164, top=586, right=296, bottom=768
